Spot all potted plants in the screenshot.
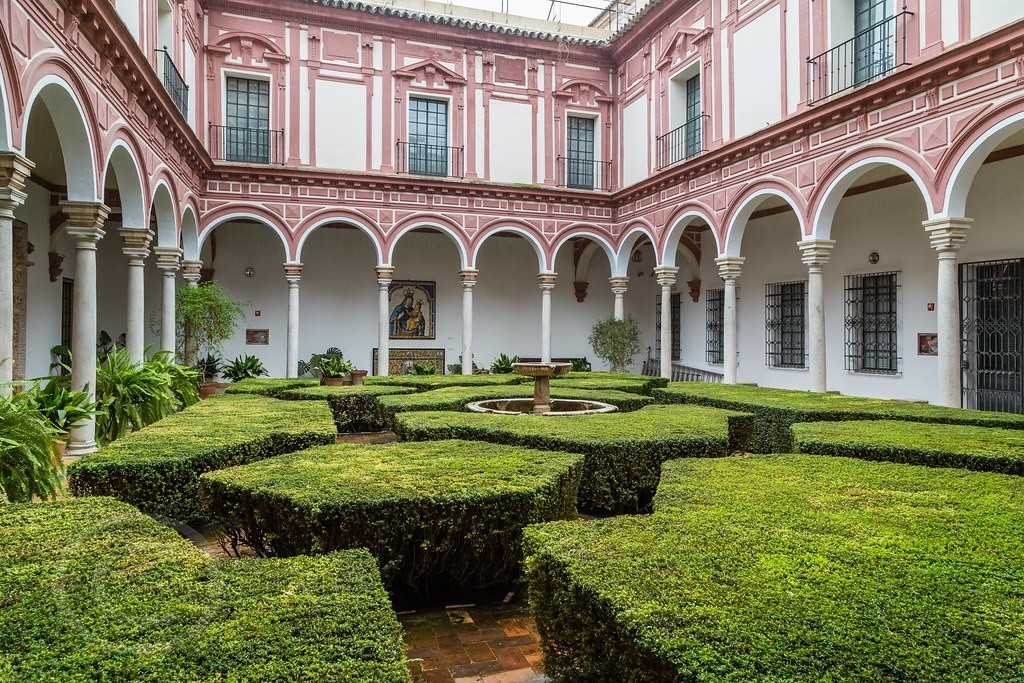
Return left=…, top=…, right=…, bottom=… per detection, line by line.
left=26, top=374, right=106, bottom=471
left=351, top=366, right=367, bottom=386
left=313, top=357, right=352, bottom=386
left=155, top=282, right=247, bottom=399
